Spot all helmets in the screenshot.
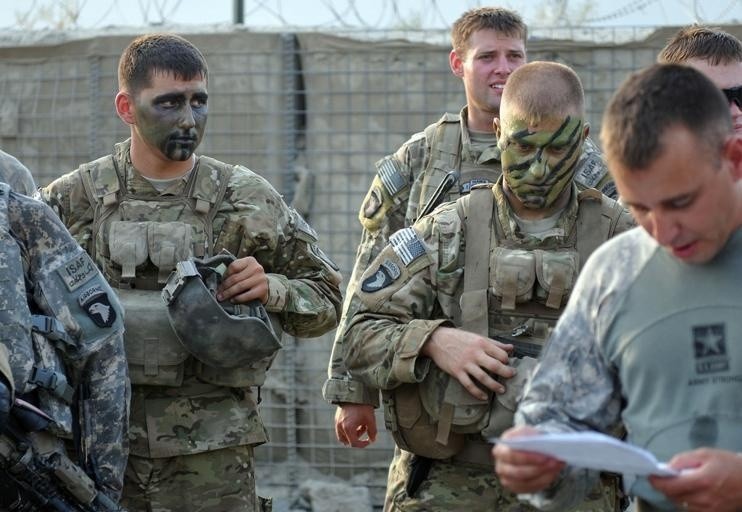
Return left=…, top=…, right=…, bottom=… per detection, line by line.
left=160, top=248, right=283, bottom=368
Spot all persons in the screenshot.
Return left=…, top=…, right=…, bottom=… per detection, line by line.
left=319, top=4, right=622, bottom=511
left=0, top=149, right=44, bottom=209
left=653, top=21, right=742, bottom=155
left=336, top=59, right=647, bottom=512
left=486, top=62, right=742, bottom=511
left=0, top=182, right=131, bottom=511
left=33, top=31, right=347, bottom=512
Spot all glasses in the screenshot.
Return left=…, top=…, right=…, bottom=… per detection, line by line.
left=721, top=86, right=742, bottom=107
